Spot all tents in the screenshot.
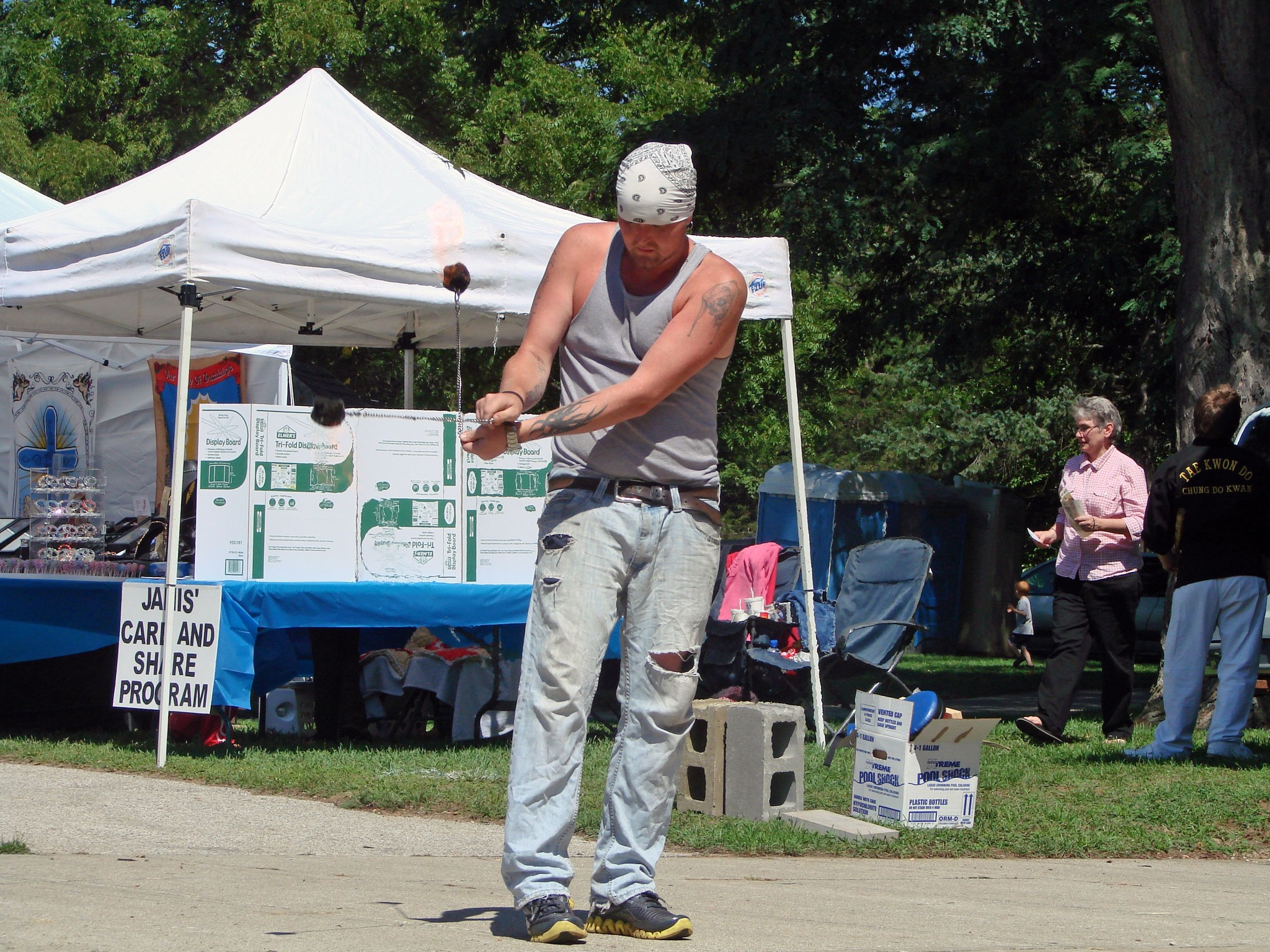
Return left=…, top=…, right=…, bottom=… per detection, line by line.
left=756, top=462, right=1019, bottom=659
left=0, top=68, right=823, bottom=768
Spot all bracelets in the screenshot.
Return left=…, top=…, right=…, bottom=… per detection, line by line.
left=502, top=391, right=524, bottom=409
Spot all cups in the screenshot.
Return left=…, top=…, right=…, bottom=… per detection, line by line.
left=731, top=609, right=744, bottom=621
left=743, top=596, right=764, bottom=615
left=754, top=610, right=773, bottom=619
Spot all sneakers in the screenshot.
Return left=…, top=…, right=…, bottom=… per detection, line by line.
left=1206, top=741, right=1256, bottom=760
left=524, top=895, right=587, bottom=942
left=1122, top=743, right=1190, bottom=759
left=584, top=891, right=693, bottom=939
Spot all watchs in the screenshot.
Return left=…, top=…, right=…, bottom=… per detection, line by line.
left=505, top=422, right=522, bottom=452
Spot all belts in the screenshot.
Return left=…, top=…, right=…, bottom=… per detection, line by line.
left=547, top=476, right=723, bottom=527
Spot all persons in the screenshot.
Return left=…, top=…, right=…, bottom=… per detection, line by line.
left=1016, top=396, right=1148, bottom=747
left=1008, top=581, right=1034, bottom=669
left=459, top=142, right=748, bottom=944
left=1125, top=384, right=1270, bottom=762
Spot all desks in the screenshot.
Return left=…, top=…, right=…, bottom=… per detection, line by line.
left=0, top=572, right=625, bottom=750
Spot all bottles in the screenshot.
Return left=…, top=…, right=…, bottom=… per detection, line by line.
left=767, top=640, right=782, bottom=654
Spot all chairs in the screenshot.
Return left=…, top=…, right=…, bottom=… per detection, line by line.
left=357, top=627, right=521, bottom=741
left=702, top=536, right=935, bottom=745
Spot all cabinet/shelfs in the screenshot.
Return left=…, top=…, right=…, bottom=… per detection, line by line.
left=30, top=468, right=105, bottom=562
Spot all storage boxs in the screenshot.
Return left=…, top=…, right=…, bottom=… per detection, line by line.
left=850, top=690, right=1010, bottom=828
left=151, top=561, right=189, bottom=577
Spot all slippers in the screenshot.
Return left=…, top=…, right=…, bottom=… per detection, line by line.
left=1102, top=736, right=1132, bottom=743
left=1016, top=716, right=1062, bottom=744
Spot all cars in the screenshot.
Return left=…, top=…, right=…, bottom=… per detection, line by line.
left=1021, top=551, right=1222, bottom=660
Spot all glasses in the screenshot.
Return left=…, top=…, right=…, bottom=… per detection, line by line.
left=1072, top=425, right=1100, bottom=435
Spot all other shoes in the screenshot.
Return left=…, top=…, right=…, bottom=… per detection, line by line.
left=1013, top=655, right=1026, bottom=666
left=1021, top=664, right=1034, bottom=669
